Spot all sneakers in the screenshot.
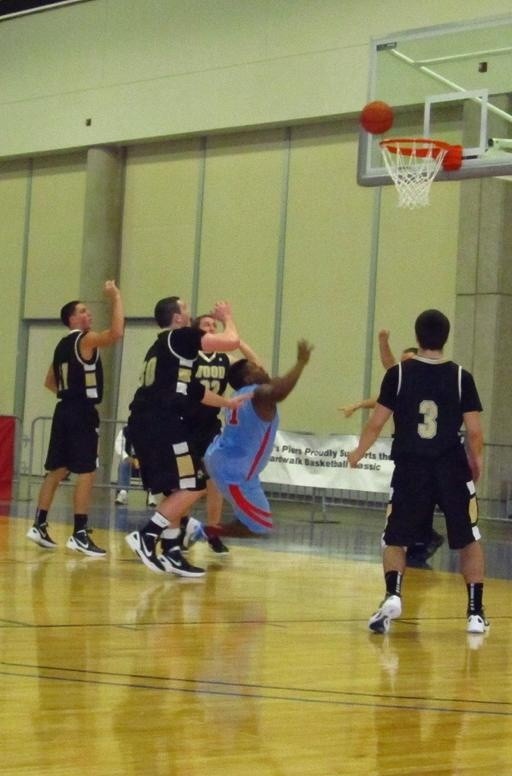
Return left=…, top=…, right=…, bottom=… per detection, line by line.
left=65, top=526, right=108, bottom=559
left=123, top=530, right=167, bottom=575
left=114, top=490, right=130, bottom=508
left=25, top=521, right=59, bottom=549
left=147, top=493, right=158, bottom=508
left=465, top=608, right=489, bottom=635
left=368, top=594, right=403, bottom=636
left=180, top=517, right=205, bottom=552
left=158, top=546, right=207, bottom=578
left=406, top=526, right=444, bottom=569
left=208, top=538, right=232, bottom=555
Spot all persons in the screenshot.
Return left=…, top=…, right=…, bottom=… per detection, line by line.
left=336, top=330, right=443, bottom=565
left=181, top=338, right=314, bottom=550
left=344, top=309, right=491, bottom=633
left=114, top=402, right=156, bottom=506
left=125, top=296, right=241, bottom=577
left=25, top=279, right=124, bottom=557
left=187, top=316, right=257, bottom=555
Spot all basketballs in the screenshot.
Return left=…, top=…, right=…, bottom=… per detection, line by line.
left=360, top=101, right=394, bottom=134
